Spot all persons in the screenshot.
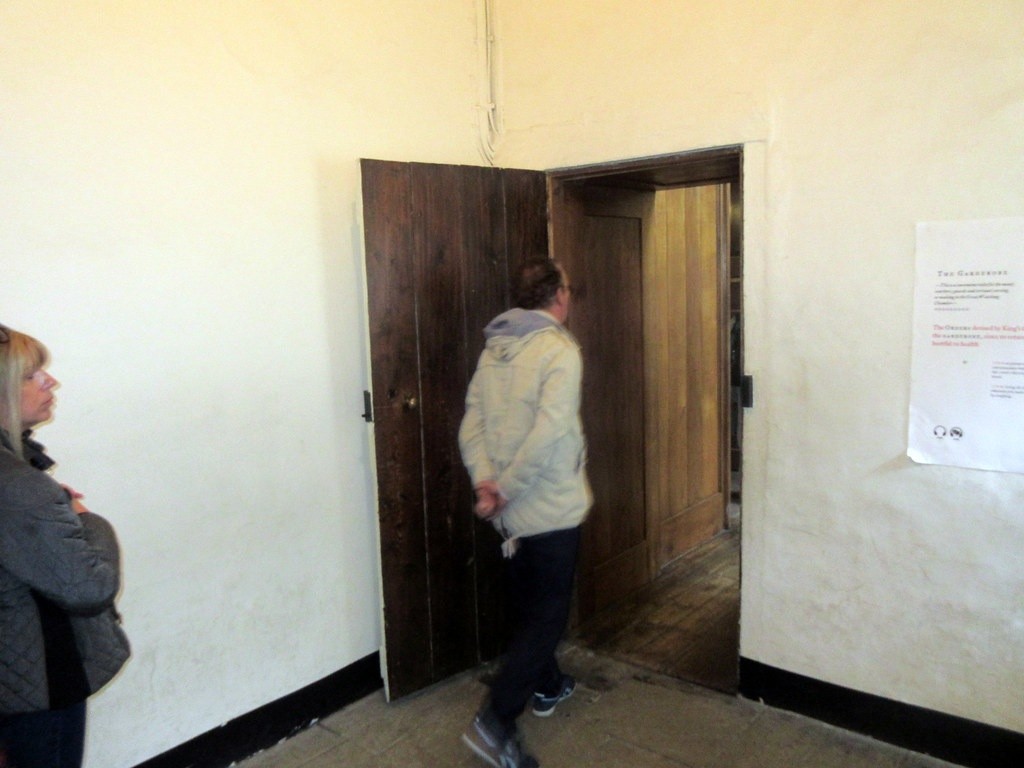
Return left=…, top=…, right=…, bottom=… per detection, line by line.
left=455, top=251, right=600, bottom=768
left=0, top=323, right=133, bottom=768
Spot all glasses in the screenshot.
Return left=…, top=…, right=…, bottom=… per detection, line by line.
left=0, top=329, right=10, bottom=343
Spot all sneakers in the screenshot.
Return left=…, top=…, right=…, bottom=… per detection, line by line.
left=459, top=712, right=538, bottom=768
left=532, top=675, right=576, bottom=717
